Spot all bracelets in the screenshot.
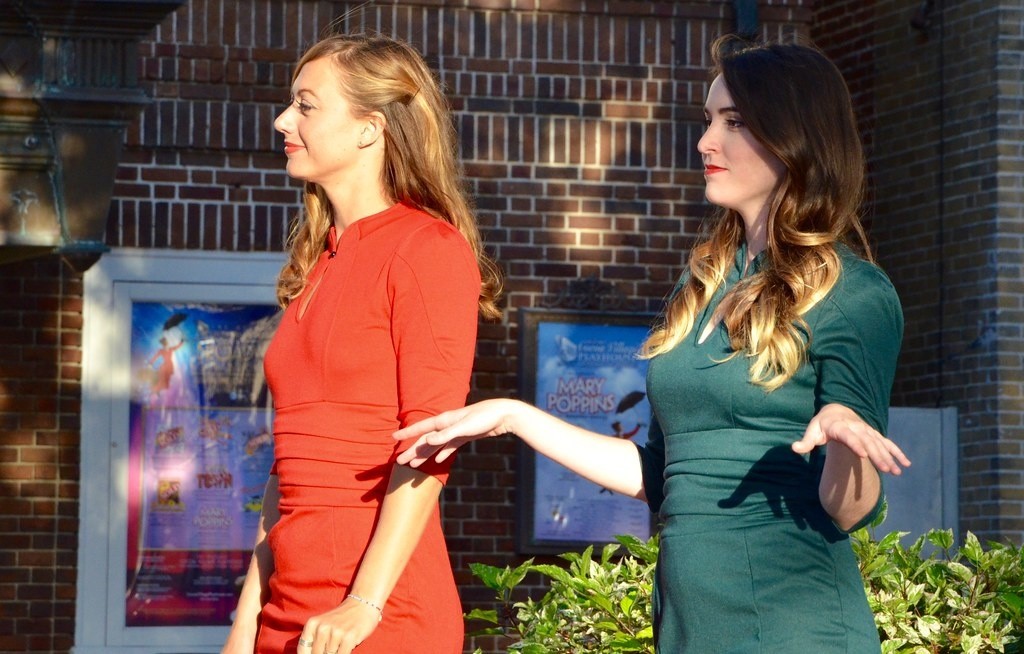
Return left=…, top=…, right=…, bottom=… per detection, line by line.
left=347, top=595, right=383, bottom=613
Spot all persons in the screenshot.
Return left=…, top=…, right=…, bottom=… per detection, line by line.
left=219, top=34, right=505, bottom=654
left=392, top=36, right=911, bottom=654
